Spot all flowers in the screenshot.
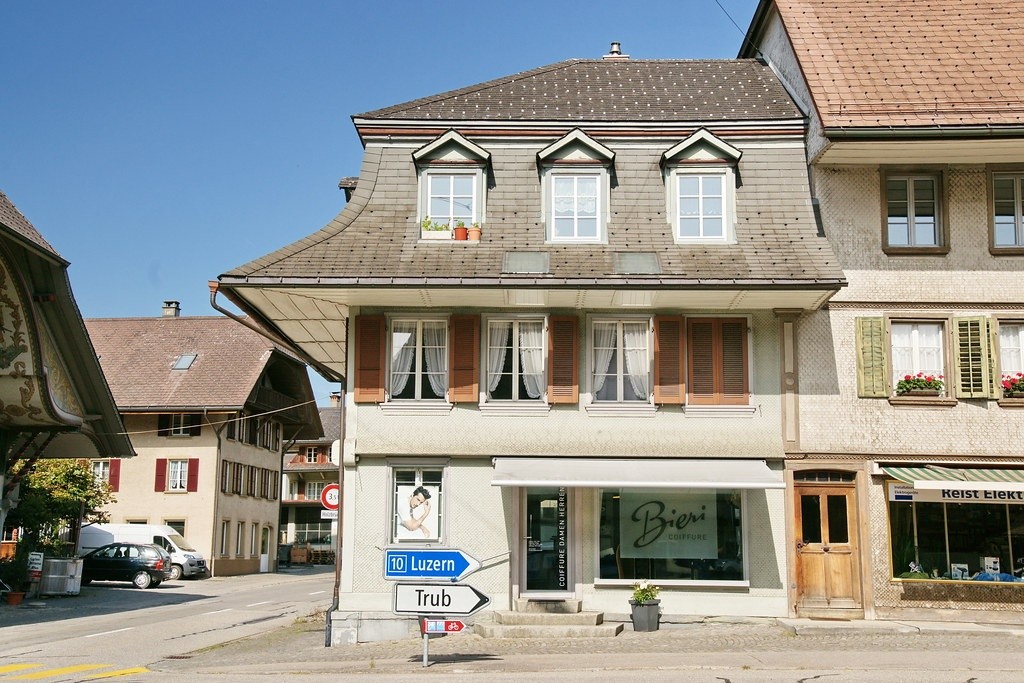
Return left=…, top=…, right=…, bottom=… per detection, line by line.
left=1000, top=372, right=1024, bottom=397
left=631, top=579, right=660, bottom=604
left=894, top=372, right=946, bottom=396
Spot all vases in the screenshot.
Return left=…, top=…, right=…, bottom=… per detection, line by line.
left=629, top=599, right=661, bottom=632
left=903, top=389, right=939, bottom=396
left=7, top=591, right=25, bottom=605
left=1010, top=391, right=1024, bottom=397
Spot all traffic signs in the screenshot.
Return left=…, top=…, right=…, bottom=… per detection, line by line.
left=422, top=619, right=466, bottom=634
left=381, top=547, right=484, bottom=582
left=392, top=583, right=491, bottom=616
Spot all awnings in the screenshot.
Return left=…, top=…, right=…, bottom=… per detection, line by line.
left=490, top=458, right=787, bottom=489
left=881, top=467, right=1024, bottom=492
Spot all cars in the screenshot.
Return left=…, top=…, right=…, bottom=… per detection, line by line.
left=78, top=542, right=172, bottom=589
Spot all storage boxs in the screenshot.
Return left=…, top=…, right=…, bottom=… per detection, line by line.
left=951, top=564, right=969, bottom=580
left=980, top=556, right=999, bottom=575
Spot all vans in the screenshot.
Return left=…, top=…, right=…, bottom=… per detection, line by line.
left=59, top=521, right=206, bottom=581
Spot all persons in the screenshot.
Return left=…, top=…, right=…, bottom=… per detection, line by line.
left=398, top=486, right=432, bottom=538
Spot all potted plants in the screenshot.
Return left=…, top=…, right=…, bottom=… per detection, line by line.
left=420, top=216, right=453, bottom=240
left=452, top=221, right=469, bottom=240
left=467, top=222, right=481, bottom=240
